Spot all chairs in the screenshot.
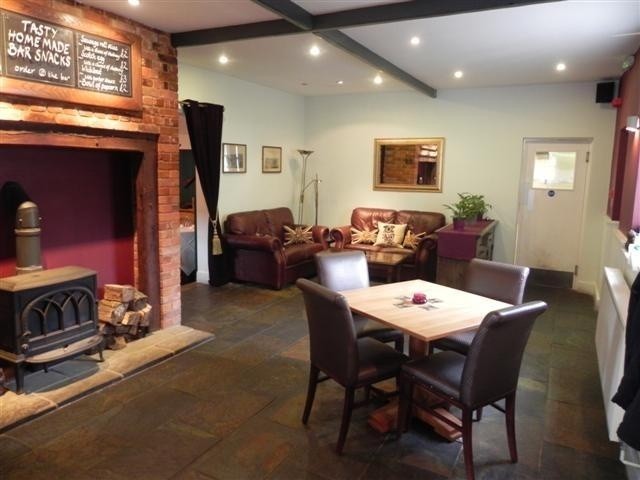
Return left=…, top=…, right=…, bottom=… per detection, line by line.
left=295, top=276, right=405, bottom=456
left=430, top=257, right=530, bottom=399
left=314, top=249, right=406, bottom=387
left=406, top=300, right=549, bottom=480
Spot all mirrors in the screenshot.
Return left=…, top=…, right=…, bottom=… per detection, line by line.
left=373, top=137, right=446, bottom=192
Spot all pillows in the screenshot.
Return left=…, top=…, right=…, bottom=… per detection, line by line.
left=376, top=218, right=409, bottom=249
left=281, top=222, right=314, bottom=246
left=349, top=221, right=379, bottom=246
left=402, top=227, right=427, bottom=251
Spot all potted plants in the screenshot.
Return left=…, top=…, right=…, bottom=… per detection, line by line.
left=444, top=201, right=470, bottom=231
left=454, top=192, right=495, bottom=223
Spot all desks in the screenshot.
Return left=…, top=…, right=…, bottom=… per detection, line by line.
left=340, top=278, right=514, bottom=442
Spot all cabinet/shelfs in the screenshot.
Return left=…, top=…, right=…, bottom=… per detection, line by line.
left=434, top=219, right=498, bottom=262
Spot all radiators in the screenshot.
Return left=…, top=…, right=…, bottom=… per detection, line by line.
left=594, top=266, right=631, bottom=444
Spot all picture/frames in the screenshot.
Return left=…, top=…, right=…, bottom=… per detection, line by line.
left=262, top=145, right=283, bottom=172
left=0, top=1, right=145, bottom=114
left=222, top=143, right=247, bottom=175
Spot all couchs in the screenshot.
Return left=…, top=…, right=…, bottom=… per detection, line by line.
left=223, top=207, right=331, bottom=290
left=329, top=206, right=445, bottom=280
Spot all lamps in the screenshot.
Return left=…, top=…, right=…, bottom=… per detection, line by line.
left=297, top=149, right=323, bottom=227
